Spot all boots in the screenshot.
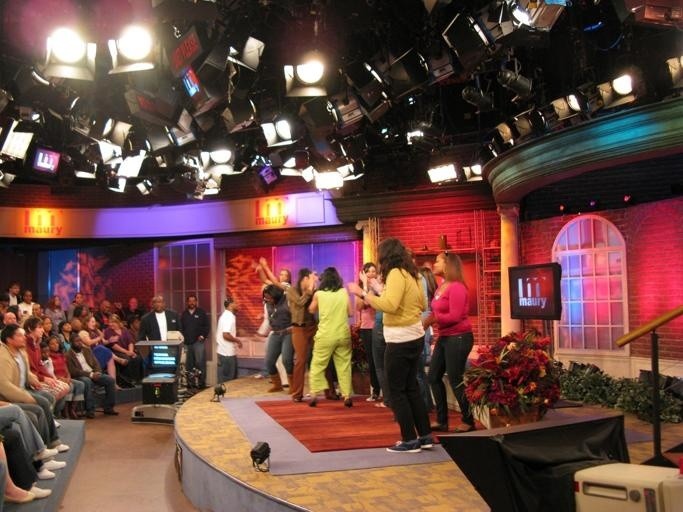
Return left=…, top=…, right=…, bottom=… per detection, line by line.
left=63, top=401, right=71, bottom=420
left=71, top=402, right=79, bottom=420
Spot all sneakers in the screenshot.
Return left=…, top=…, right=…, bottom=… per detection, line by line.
left=2, top=443, right=69, bottom=502
left=268, top=375, right=474, bottom=454
left=104, top=407, right=118, bottom=415
left=86, top=408, right=95, bottom=419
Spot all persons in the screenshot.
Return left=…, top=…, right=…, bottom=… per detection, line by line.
left=0, top=281, right=149, bottom=511
left=249, top=236, right=477, bottom=451
left=215, top=297, right=243, bottom=384
left=37, top=154, right=54, bottom=170
left=180, top=296, right=210, bottom=389
left=135, top=295, right=183, bottom=367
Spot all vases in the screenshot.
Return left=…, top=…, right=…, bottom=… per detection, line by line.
left=513, top=405, right=540, bottom=424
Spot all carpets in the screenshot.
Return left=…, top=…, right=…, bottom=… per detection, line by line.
left=254, top=395, right=486, bottom=454
left=220, top=394, right=663, bottom=474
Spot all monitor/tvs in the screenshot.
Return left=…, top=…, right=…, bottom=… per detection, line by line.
left=180, top=63, right=211, bottom=106
left=508, top=263, right=562, bottom=320
left=151, top=345, right=180, bottom=371
left=31, top=145, right=62, bottom=177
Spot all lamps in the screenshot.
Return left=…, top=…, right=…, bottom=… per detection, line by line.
left=211, top=384, right=226, bottom=403
left=0, top=0, right=683, bottom=202
left=251, top=443, right=272, bottom=474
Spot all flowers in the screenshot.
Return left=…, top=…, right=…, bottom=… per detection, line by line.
left=350, top=324, right=371, bottom=377
left=456, top=332, right=560, bottom=425
